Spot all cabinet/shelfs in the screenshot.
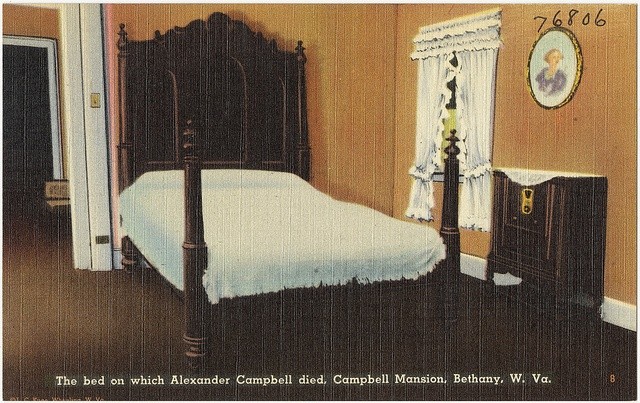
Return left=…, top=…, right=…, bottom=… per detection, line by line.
left=486, top=166, right=608, bottom=329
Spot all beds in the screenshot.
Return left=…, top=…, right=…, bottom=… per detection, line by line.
left=115, top=11, right=462, bottom=368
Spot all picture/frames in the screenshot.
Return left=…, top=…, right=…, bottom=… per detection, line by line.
left=522, top=27, right=584, bottom=110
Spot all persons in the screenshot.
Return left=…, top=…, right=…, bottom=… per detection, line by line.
left=534, top=48, right=569, bottom=99
